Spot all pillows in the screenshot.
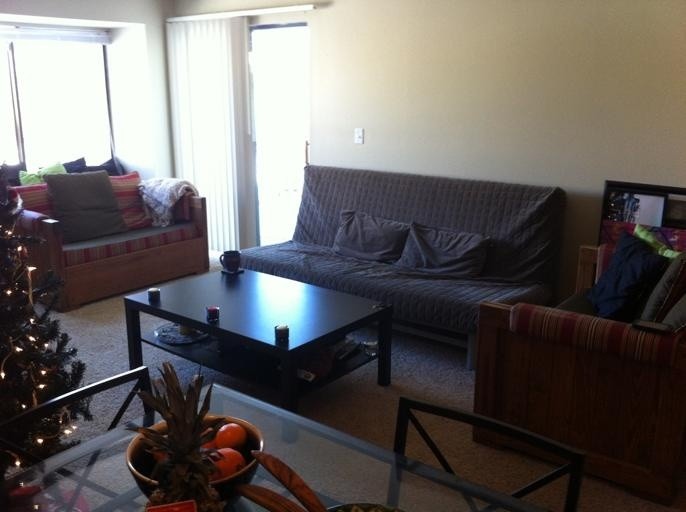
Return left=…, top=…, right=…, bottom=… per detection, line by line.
left=331, top=210, right=414, bottom=261
left=392, top=225, right=491, bottom=284
left=589, top=224, right=685, bottom=336
left=42, top=170, right=127, bottom=243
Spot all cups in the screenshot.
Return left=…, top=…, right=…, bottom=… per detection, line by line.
left=147, top=288, right=160, bottom=304
left=220, top=251, right=240, bottom=271
left=275, top=326, right=290, bottom=344
left=205, top=305, right=220, bottom=322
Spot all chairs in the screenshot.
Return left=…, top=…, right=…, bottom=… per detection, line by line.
left=389, top=396, right=586, bottom=508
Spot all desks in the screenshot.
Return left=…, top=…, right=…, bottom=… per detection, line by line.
left=0, top=380, right=537, bottom=512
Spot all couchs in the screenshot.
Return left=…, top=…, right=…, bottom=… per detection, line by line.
left=471, top=222, right=686, bottom=511
left=7, top=170, right=209, bottom=312
left=229, top=162, right=566, bottom=374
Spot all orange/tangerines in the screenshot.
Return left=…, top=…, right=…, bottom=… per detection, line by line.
left=153, top=423, right=247, bottom=480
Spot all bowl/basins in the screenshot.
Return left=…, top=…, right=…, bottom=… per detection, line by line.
left=126, top=414, right=264, bottom=507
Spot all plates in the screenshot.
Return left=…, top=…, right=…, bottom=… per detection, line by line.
left=152, top=322, right=208, bottom=345
left=221, top=270, right=245, bottom=274
